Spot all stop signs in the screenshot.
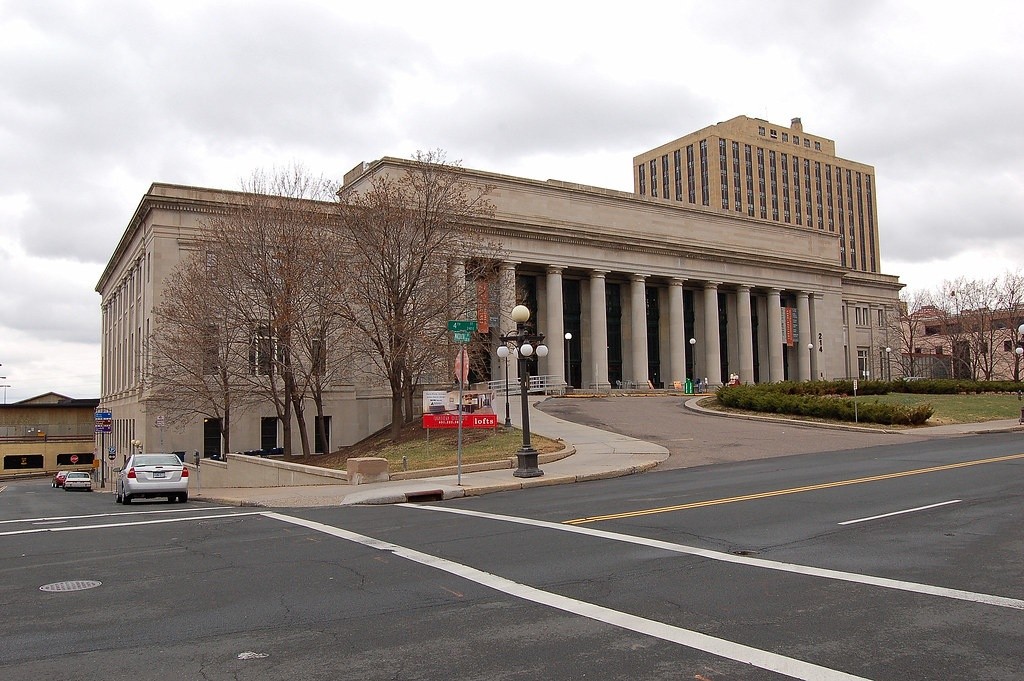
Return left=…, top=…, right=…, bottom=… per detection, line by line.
left=70, top=455, right=79, bottom=464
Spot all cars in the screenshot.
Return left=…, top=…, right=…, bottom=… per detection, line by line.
left=51, top=471, right=68, bottom=488
left=113, top=452, right=190, bottom=504
left=63, top=471, right=92, bottom=491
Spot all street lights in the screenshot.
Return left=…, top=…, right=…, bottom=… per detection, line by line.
left=808, top=343, right=814, bottom=381
left=690, top=338, right=697, bottom=383
left=886, top=347, right=892, bottom=382
left=496, top=305, right=550, bottom=478
left=565, top=332, right=575, bottom=389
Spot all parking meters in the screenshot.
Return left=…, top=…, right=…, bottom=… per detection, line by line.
left=192, top=448, right=200, bottom=494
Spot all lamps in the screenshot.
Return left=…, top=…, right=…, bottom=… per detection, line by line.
left=135, top=440, right=143, bottom=449
left=131, top=440, right=139, bottom=449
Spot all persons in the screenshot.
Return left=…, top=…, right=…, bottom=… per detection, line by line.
left=727, top=372, right=739, bottom=384
